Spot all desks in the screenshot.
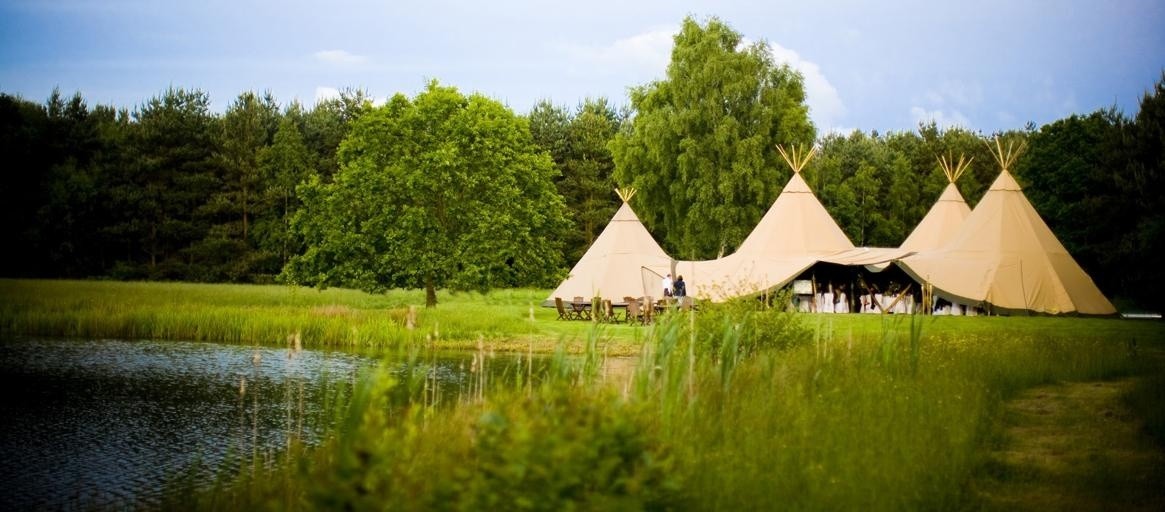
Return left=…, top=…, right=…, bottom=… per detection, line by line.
left=556, top=296, right=700, bottom=326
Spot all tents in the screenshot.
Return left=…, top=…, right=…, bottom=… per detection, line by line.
left=546, top=201, right=674, bottom=307
left=897, top=171, right=1123, bottom=319
left=676, top=174, right=920, bottom=306
left=898, top=180, right=973, bottom=253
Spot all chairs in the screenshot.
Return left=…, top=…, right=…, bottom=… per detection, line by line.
left=799, top=292, right=978, bottom=317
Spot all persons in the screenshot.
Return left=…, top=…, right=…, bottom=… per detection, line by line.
left=674, top=275, right=686, bottom=301
left=663, top=273, right=674, bottom=297
